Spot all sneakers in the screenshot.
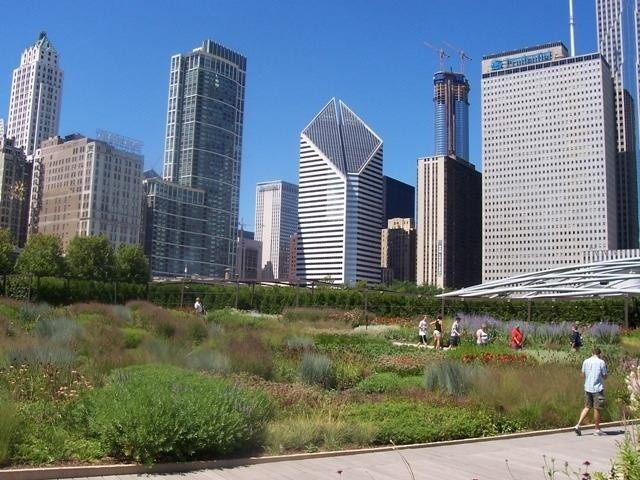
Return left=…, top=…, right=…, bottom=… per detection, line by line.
left=593, top=429, right=607, bottom=436
left=574, top=424, right=581, bottom=436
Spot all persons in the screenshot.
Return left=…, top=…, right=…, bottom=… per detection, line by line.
left=416, top=314, right=429, bottom=349
left=511, top=322, right=526, bottom=351
left=194, top=297, right=203, bottom=316
left=450, top=317, right=462, bottom=346
left=570, top=325, right=581, bottom=351
left=573, top=348, right=607, bottom=436
left=428, top=315, right=444, bottom=348
left=475, top=322, right=488, bottom=346
